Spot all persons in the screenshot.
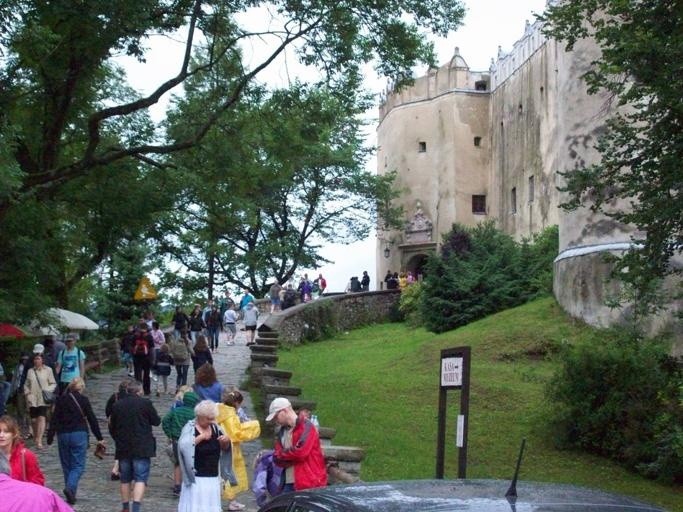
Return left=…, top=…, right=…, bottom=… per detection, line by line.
left=263, top=397, right=326, bottom=492
left=298, top=406, right=313, bottom=420
left=1, top=290, right=262, bottom=512
left=267, top=264, right=418, bottom=315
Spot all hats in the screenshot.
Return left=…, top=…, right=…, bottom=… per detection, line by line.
left=64, top=335, right=75, bottom=341
left=264, top=398, right=291, bottom=422
left=33, top=344, right=45, bottom=354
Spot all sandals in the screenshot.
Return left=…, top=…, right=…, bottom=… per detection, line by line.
left=24, top=432, right=32, bottom=439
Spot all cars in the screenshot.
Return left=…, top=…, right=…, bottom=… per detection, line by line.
left=258, top=436, right=674, bottom=512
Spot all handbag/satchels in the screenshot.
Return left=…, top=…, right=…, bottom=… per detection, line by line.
left=42, top=390, right=55, bottom=404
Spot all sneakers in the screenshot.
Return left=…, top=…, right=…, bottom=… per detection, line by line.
left=174, top=485, right=182, bottom=494
left=229, top=501, right=245, bottom=510
left=129, top=340, right=256, bottom=396
left=63, top=488, right=75, bottom=504
left=111, top=473, right=118, bottom=480
left=36, top=441, right=43, bottom=450
